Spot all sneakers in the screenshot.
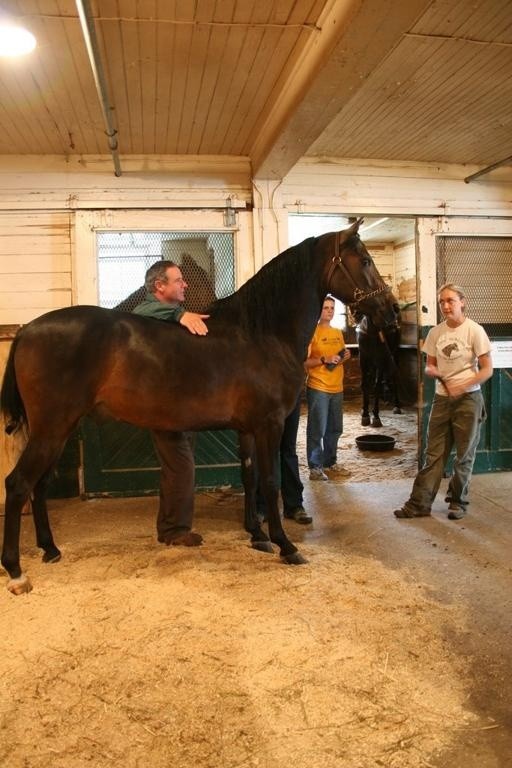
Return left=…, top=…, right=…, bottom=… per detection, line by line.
left=294, top=511, right=314, bottom=525
left=446, top=503, right=467, bottom=520
left=309, top=462, right=352, bottom=482
left=156, top=531, right=204, bottom=547
left=393, top=506, right=433, bottom=518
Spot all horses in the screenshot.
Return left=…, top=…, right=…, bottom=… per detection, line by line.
left=0, top=215, right=403, bottom=596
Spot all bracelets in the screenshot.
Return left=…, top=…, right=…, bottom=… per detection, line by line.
left=321, top=356, right=325, bottom=364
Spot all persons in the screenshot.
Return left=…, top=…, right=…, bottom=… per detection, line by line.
left=131, top=259, right=211, bottom=546
left=255, top=398, right=314, bottom=524
left=305, top=297, right=351, bottom=482
left=394, top=283, right=493, bottom=519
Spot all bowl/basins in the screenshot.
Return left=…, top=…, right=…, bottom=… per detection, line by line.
left=355, top=434, right=396, bottom=450
